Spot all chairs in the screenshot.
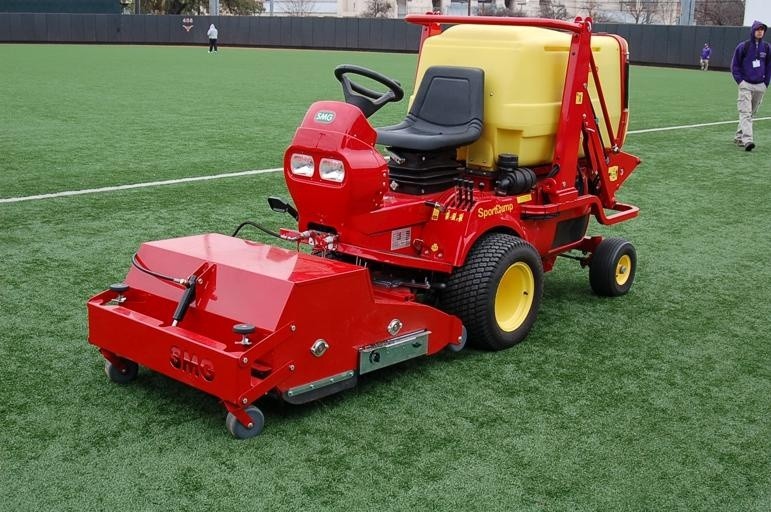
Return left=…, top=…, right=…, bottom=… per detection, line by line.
left=373, top=64, right=485, bottom=151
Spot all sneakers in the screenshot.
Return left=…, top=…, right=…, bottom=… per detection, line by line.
left=734, top=136, right=755, bottom=151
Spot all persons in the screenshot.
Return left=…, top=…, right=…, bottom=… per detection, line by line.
left=730, top=20, right=771, bottom=151
left=206, top=23, right=219, bottom=54
left=697, top=42, right=712, bottom=71
